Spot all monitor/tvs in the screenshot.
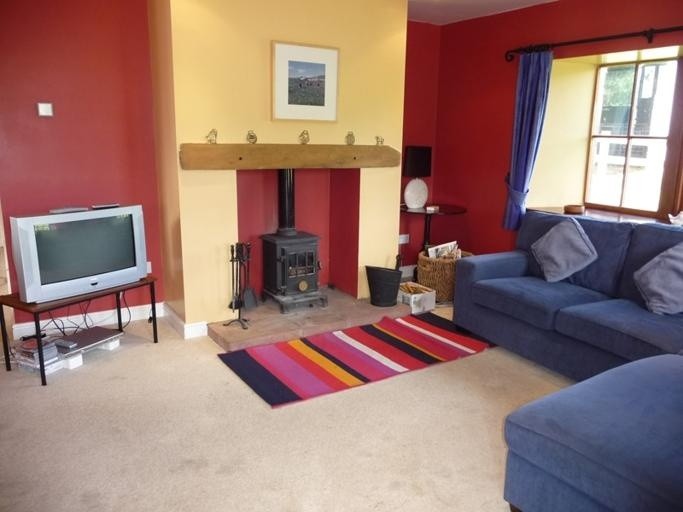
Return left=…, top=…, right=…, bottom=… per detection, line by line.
left=10, top=206, right=148, bottom=304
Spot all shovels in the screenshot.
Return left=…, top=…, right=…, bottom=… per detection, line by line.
left=243, top=241, right=257, bottom=311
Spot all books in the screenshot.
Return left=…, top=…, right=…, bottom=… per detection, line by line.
left=427, top=238, right=458, bottom=259
left=423, top=244, right=435, bottom=257
left=12, top=336, right=64, bottom=377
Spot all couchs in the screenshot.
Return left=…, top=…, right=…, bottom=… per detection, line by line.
left=453, top=207, right=683, bottom=381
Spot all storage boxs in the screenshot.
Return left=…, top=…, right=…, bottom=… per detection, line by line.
left=399, top=282, right=436, bottom=316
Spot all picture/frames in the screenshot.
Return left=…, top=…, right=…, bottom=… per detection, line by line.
left=271, top=39, right=337, bottom=122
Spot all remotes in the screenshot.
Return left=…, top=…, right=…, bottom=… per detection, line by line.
left=56, top=337, right=78, bottom=349
left=48, top=208, right=88, bottom=213
left=90, top=202, right=120, bottom=209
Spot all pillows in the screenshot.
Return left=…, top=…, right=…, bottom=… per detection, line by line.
left=631, top=240, right=683, bottom=317
left=530, top=217, right=600, bottom=283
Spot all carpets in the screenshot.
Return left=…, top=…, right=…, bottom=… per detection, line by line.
left=219, top=313, right=498, bottom=407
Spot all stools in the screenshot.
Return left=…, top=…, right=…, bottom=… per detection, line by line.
left=503, top=352, right=682, bottom=511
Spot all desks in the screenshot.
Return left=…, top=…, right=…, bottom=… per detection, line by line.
left=402, top=203, right=466, bottom=249
left=0, top=276, right=158, bottom=385
